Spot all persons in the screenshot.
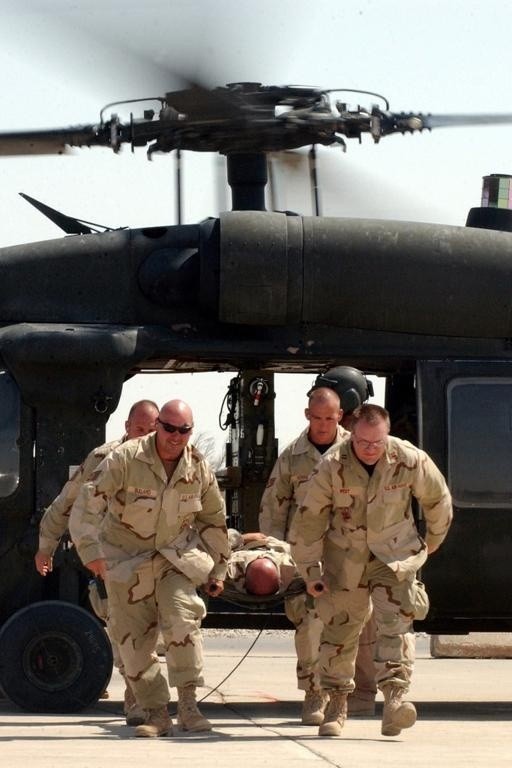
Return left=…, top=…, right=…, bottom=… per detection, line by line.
left=218, top=523, right=293, bottom=599
left=29, top=400, right=161, bottom=727
left=67, top=397, right=234, bottom=738
left=260, top=388, right=383, bottom=724
left=289, top=399, right=455, bottom=738
left=313, top=367, right=380, bottom=432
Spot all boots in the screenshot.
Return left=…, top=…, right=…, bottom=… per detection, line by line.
left=122, top=687, right=417, bottom=736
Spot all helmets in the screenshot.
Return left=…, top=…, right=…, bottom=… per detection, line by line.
left=308, top=367, right=374, bottom=412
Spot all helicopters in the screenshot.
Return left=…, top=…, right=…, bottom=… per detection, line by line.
left=0, top=0, right=512, bottom=714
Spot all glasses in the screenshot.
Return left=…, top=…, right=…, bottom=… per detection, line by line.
left=156, top=417, right=193, bottom=434
